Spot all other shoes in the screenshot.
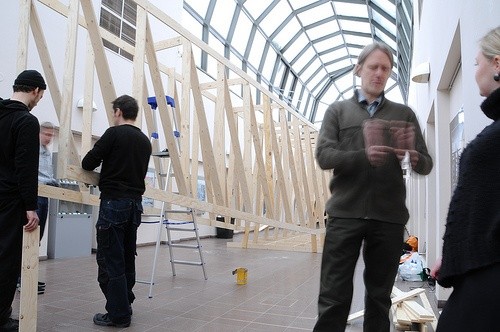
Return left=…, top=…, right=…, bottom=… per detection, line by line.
left=129, top=304, right=132, bottom=315
left=38, top=289, right=44, bottom=295
left=38, top=281, right=45, bottom=286
left=94, top=313, right=130, bottom=327
left=0, top=318, right=19, bottom=332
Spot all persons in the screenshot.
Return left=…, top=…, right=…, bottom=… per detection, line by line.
left=429, top=26, right=500, bottom=331
left=17, top=122, right=60, bottom=295
left=313, top=42, right=433, bottom=332
left=0, top=70, right=47, bottom=332
left=82, top=95, right=153, bottom=327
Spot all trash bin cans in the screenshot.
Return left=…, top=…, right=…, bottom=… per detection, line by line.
left=216, top=217, right=235, bottom=239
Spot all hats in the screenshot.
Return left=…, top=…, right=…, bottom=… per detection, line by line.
left=15, top=70, right=46, bottom=89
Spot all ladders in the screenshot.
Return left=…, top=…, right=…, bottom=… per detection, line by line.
left=135, top=95, right=209, bottom=299
